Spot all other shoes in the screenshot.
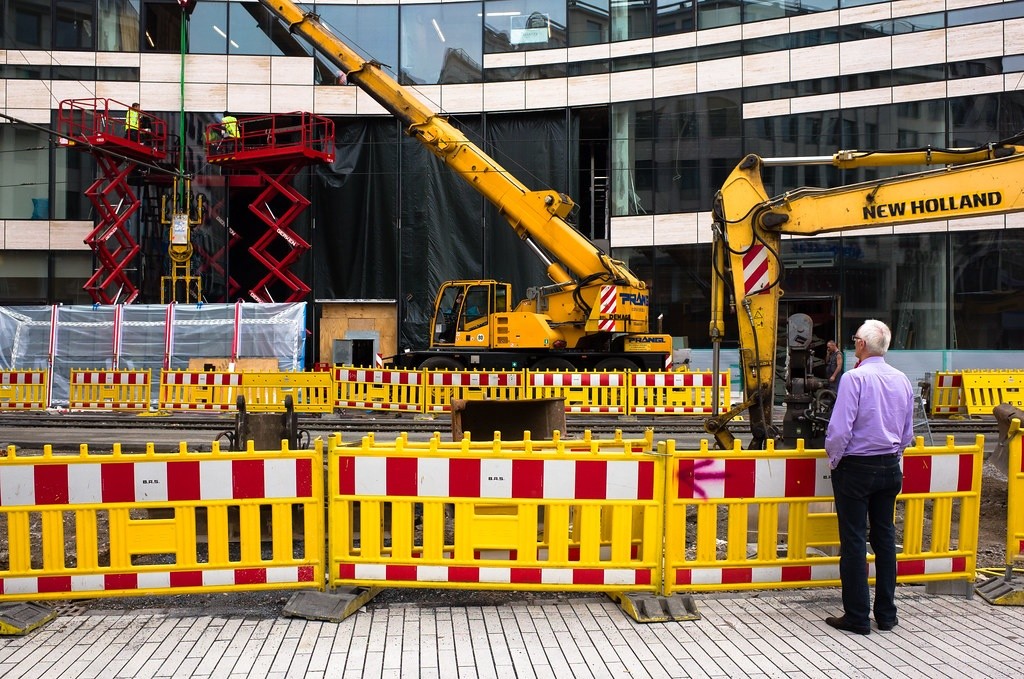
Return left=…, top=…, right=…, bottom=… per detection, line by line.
left=877, top=617, right=898, bottom=630
left=826, top=616, right=870, bottom=635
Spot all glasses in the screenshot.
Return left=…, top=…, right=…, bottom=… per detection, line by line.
left=852, top=335, right=866, bottom=344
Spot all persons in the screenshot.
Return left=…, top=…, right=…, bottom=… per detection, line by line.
left=826, top=340, right=844, bottom=393
left=824, top=317, right=913, bottom=636
left=201, top=122, right=222, bottom=155
left=125, top=103, right=141, bottom=143
left=216, top=111, right=242, bottom=152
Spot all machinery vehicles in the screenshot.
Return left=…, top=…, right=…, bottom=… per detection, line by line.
left=706, top=132, right=1024, bottom=451
left=258, top=0, right=673, bottom=374
